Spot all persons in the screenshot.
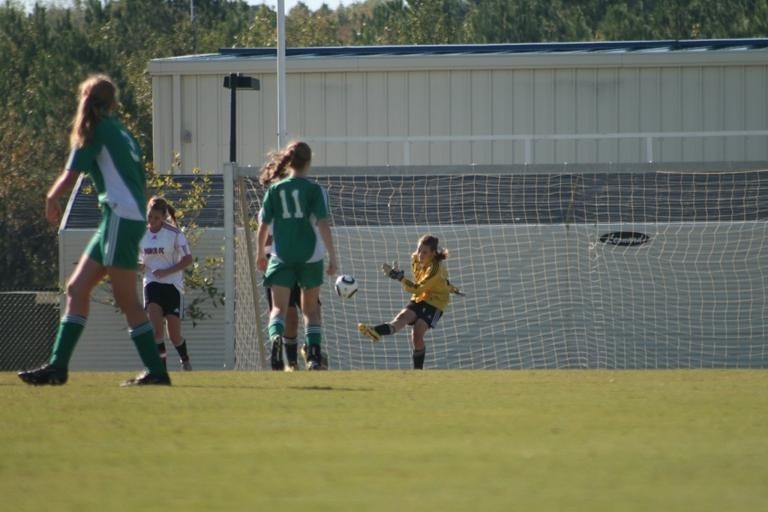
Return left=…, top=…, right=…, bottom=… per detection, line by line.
left=354, top=234, right=467, bottom=371
left=134, top=194, right=196, bottom=374
left=12, top=70, right=174, bottom=389
left=258, top=160, right=330, bottom=372
left=251, top=137, right=338, bottom=375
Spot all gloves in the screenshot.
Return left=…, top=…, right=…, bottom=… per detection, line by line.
left=380, top=260, right=404, bottom=282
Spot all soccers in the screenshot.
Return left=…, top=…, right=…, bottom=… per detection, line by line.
left=334, top=274, right=358, bottom=299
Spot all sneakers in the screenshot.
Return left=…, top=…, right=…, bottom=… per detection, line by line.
left=120, top=367, right=171, bottom=387
left=265, top=335, right=321, bottom=370
left=180, top=354, right=193, bottom=371
left=356, top=322, right=381, bottom=343
left=16, top=362, right=69, bottom=386
